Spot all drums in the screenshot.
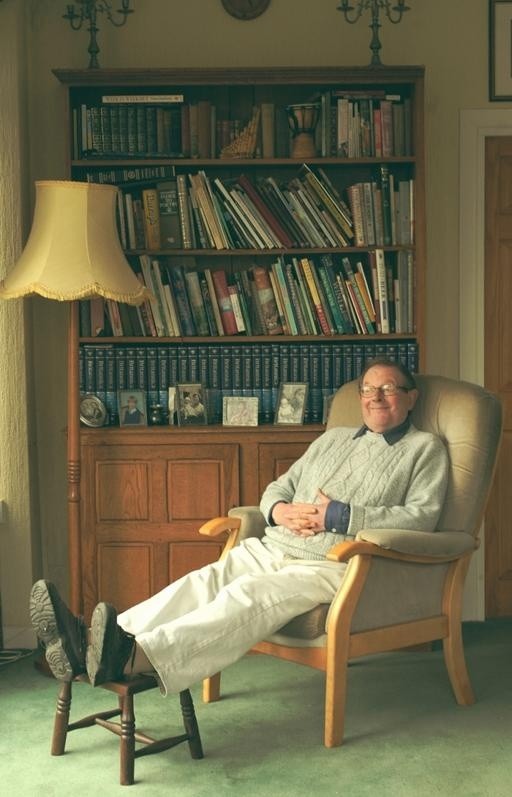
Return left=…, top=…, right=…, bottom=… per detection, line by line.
left=285, top=103, right=321, bottom=158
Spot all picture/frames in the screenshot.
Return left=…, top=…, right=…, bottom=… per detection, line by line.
left=485, top=1, right=511, bottom=106
left=80, top=380, right=312, bottom=429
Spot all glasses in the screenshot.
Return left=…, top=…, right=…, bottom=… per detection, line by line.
left=359, top=384, right=405, bottom=398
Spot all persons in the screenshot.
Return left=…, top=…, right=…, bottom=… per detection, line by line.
left=184, top=393, right=205, bottom=425
left=29, top=357, right=451, bottom=699
left=230, top=402, right=251, bottom=425
left=122, top=396, right=141, bottom=424
left=91, top=402, right=102, bottom=422
left=278, top=388, right=305, bottom=424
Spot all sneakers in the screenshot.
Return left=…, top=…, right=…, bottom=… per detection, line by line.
left=85, top=600, right=136, bottom=687
left=28, top=578, right=89, bottom=682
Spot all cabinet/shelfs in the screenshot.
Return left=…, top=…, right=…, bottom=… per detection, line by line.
left=65, top=83, right=428, bottom=625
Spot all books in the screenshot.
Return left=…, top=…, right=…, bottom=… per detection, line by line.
left=178, top=347, right=187, bottom=384
left=209, top=344, right=417, bottom=429
left=189, top=347, right=198, bottom=384
left=72, top=249, right=417, bottom=339
left=72, top=87, right=415, bottom=160
left=73, top=344, right=178, bottom=427
left=199, top=346, right=209, bottom=424
left=72, top=165, right=415, bottom=250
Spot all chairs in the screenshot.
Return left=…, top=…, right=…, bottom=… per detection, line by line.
left=199, top=368, right=505, bottom=750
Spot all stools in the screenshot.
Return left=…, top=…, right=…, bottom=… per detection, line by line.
left=34, top=654, right=204, bottom=785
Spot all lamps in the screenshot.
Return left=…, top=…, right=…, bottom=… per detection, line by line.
left=0, top=179, right=151, bottom=612
